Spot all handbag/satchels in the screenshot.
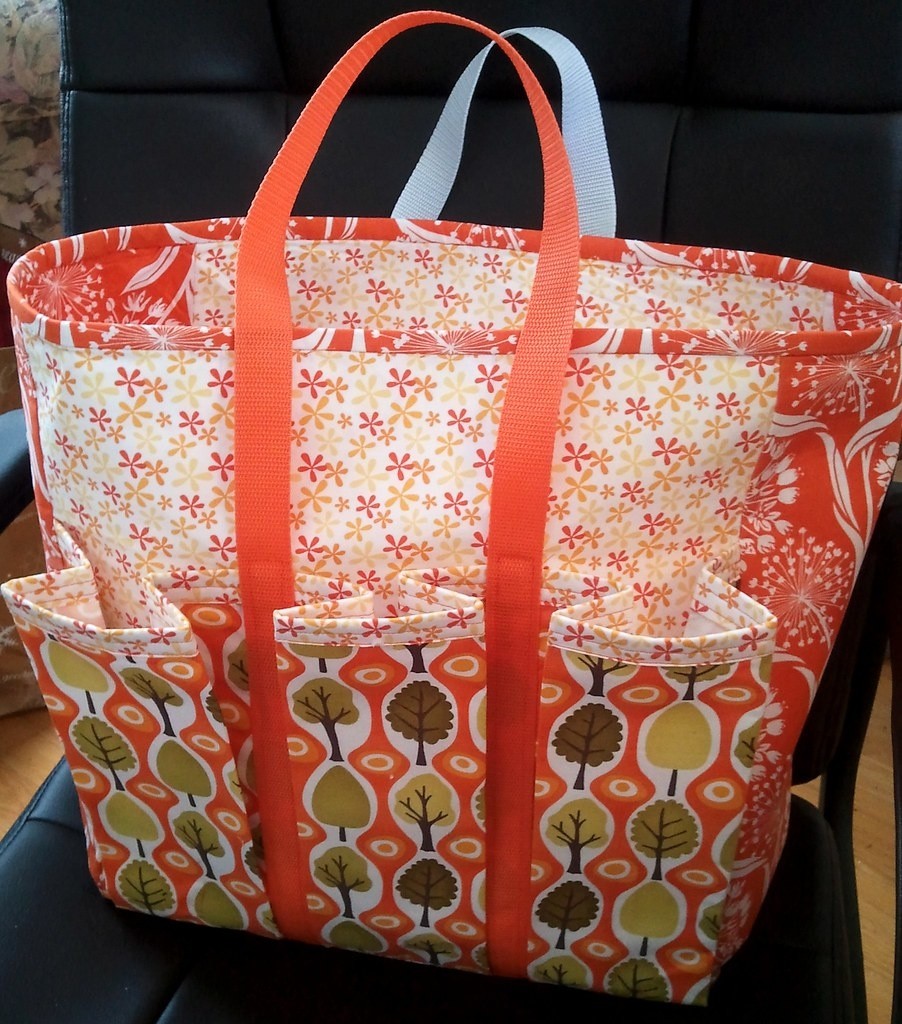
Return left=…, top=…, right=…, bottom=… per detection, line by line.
left=1, top=10, right=902, bottom=1008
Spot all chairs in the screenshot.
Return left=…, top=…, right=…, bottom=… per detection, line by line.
left=0, top=0, right=902, bottom=1024
